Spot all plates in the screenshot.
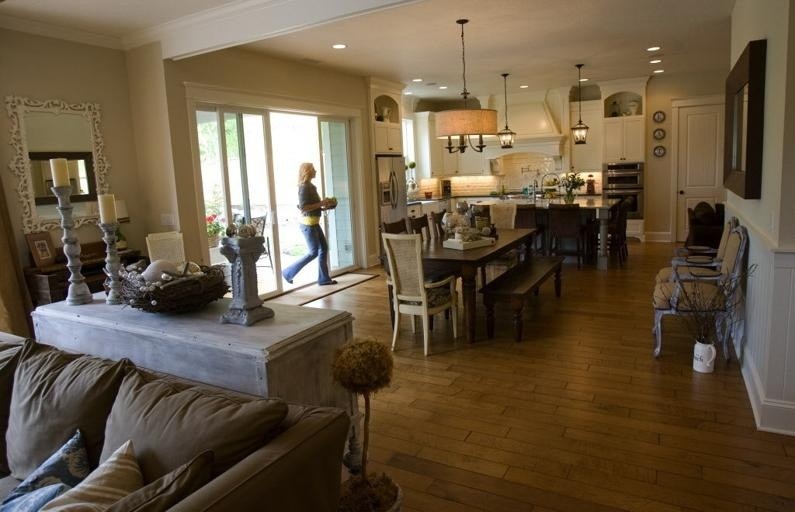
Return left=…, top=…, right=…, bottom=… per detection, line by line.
left=653, top=110, right=666, bottom=157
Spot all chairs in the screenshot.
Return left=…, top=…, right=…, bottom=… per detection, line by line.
left=407, top=214, right=449, bottom=319
left=653, top=216, right=748, bottom=360
left=547, top=203, right=584, bottom=271
left=381, top=233, right=458, bottom=357
left=378, top=218, right=433, bottom=332
left=485, top=203, right=516, bottom=266
left=584, top=196, right=634, bottom=265
left=431, top=209, right=446, bottom=238
left=515, top=204, right=546, bottom=251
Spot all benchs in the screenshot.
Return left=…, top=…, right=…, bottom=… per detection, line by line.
left=478, top=256, right=565, bottom=342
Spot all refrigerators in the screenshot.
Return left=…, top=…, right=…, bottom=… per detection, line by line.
left=376, top=157, right=409, bottom=265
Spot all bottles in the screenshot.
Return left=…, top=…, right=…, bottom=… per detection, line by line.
left=609, top=100, right=640, bottom=116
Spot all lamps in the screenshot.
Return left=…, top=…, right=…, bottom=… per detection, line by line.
left=570, top=64, right=589, bottom=144
left=436, top=19, right=497, bottom=154
left=497, top=73, right=515, bottom=148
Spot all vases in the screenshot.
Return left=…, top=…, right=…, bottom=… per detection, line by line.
left=693, top=339, right=716, bottom=373
left=208, top=237, right=220, bottom=247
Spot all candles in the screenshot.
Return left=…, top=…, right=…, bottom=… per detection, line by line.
left=97, top=194, right=116, bottom=223
left=50, top=158, right=70, bottom=188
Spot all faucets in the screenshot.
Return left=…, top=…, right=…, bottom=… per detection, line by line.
left=533, top=179, right=539, bottom=201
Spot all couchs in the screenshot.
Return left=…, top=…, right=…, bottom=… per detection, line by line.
left=0, top=332, right=350, bottom=512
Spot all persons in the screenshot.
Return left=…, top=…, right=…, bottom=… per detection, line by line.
left=282, top=161, right=339, bottom=285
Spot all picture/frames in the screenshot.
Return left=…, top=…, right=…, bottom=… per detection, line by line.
left=25, top=232, right=56, bottom=267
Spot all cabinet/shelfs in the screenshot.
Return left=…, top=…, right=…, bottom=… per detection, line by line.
left=605, top=116, right=644, bottom=163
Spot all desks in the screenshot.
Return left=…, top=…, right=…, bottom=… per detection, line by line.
left=415, top=228, right=537, bottom=343
left=24, top=249, right=140, bottom=304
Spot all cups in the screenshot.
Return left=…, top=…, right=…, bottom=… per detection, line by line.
left=425, top=192, right=432, bottom=200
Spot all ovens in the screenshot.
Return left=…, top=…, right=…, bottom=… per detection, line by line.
left=602, top=162, right=644, bottom=219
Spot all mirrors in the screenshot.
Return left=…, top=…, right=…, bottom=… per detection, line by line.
left=6, top=93, right=110, bottom=234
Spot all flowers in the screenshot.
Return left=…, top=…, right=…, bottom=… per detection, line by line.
left=205, top=214, right=225, bottom=236
